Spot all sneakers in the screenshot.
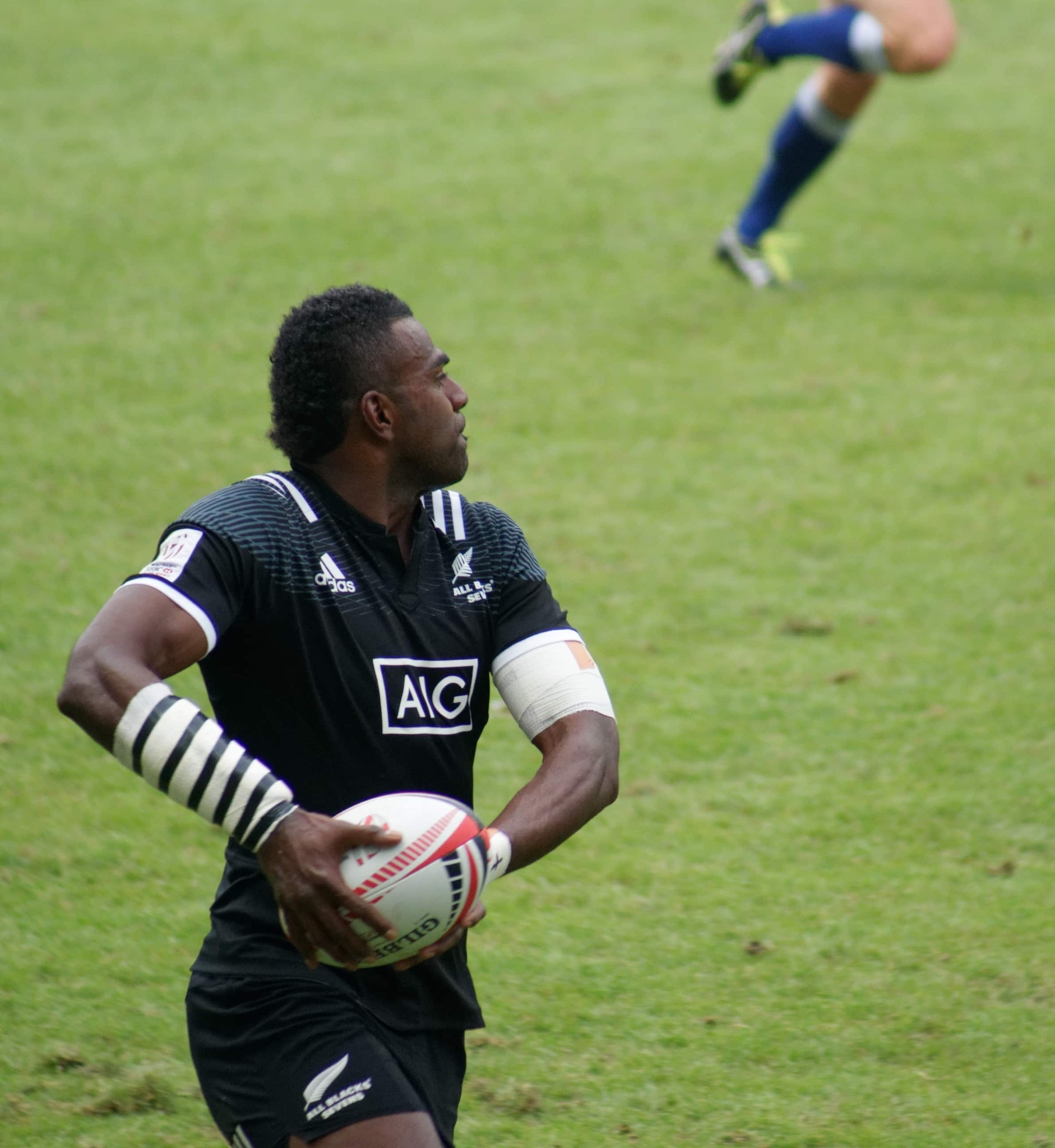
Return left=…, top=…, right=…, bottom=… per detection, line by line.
left=710, top=0, right=787, bottom=105
left=714, top=229, right=775, bottom=288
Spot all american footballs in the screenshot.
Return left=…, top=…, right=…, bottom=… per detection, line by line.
left=278, top=792, right=493, bottom=971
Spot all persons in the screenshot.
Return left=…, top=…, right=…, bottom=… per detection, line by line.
left=59, top=282, right=620, bottom=1148
left=708, top=0, right=956, bottom=292
